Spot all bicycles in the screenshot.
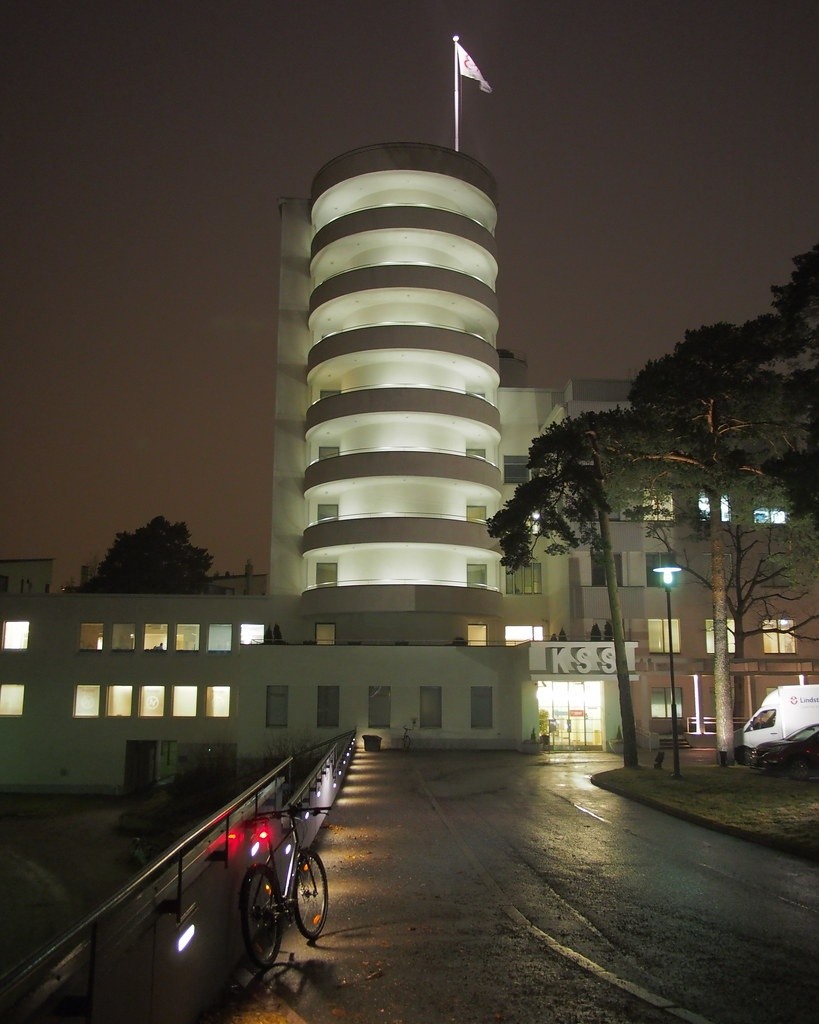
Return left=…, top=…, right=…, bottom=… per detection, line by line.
left=238, top=806, right=333, bottom=970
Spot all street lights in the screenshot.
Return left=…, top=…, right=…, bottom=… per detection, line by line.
left=652, top=561, right=683, bottom=777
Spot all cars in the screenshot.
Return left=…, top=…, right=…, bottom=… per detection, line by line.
left=751, top=723, right=819, bottom=780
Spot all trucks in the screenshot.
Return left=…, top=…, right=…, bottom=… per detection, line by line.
left=732, top=683, right=819, bottom=766
